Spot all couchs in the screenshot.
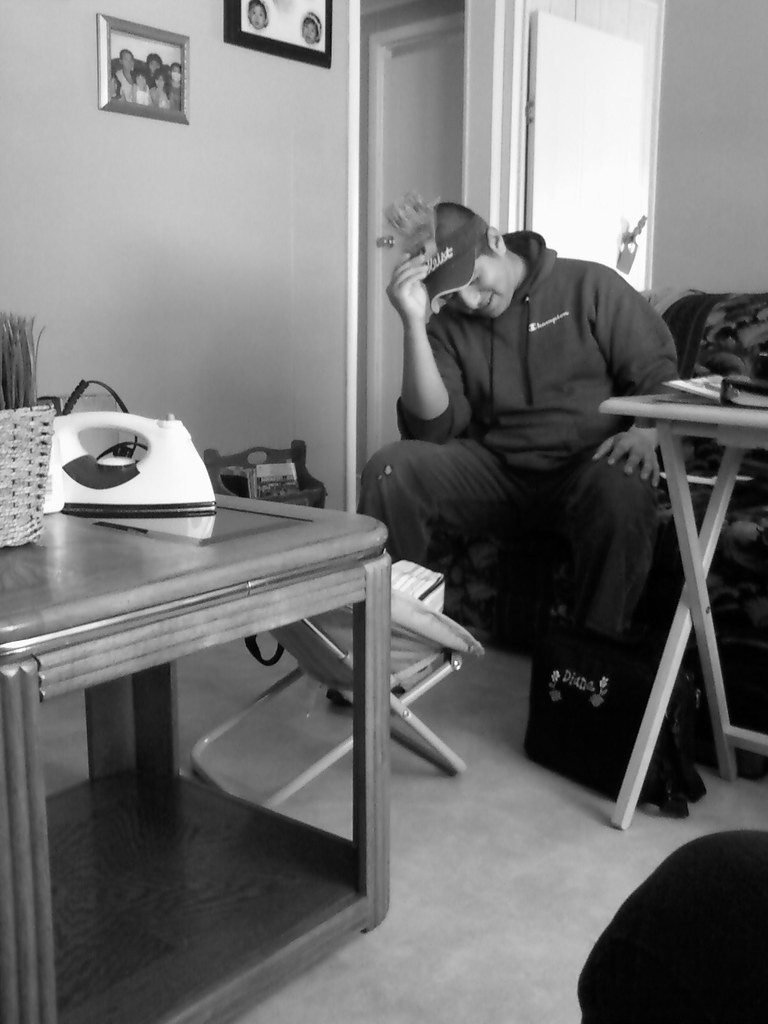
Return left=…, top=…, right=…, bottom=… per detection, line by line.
left=428, top=286, right=768, bottom=692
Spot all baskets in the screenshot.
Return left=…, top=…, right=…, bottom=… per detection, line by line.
left=0, top=401, right=57, bottom=549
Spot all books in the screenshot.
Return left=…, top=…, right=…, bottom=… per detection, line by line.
left=720, top=374, right=768, bottom=409
left=244, top=459, right=302, bottom=502
left=649, top=373, right=728, bottom=410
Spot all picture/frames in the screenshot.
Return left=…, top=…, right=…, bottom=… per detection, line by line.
left=223, top=0, right=332, bottom=70
left=95, top=12, right=189, bottom=125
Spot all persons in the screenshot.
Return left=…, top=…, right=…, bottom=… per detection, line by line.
left=319, top=202, right=693, bottom=715
left=247, top=0, right=271, bottom=30
left=299, top=10, right=323, bottom=45
left=109, top=49, right=182, bottom=112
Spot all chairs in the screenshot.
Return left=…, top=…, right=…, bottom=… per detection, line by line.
left=192, top=589, right=485, bottom=811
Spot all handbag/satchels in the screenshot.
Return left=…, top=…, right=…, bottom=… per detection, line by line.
left=577, top=830, right=768, bottom=1024
left=524, top=629, right=705, bottom=817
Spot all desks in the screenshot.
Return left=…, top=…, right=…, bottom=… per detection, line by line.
left=0, top=494, right=395, bottom=1023
left=596, top=390, right=767, bottom=831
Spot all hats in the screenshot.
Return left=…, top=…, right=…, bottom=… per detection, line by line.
left=423, top=202, right=476, bottom=314
